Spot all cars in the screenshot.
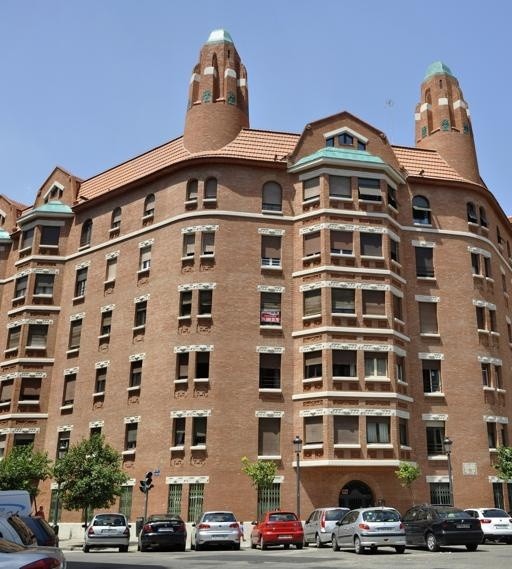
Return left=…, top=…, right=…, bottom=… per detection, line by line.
left=331, top=506, right=407, bottom=554
left=249, top=511, right=304, bottom=550
left=0, top=490, right=70, bottom=569
left=81, top=513, right=131, bottom=552
left=135, top=514, right=187, bottom=552
left=191, top=511, right=241, bottom=551
left=401, top=504, right=484, bottom=552
left=464, top=508, right=512, bottom=545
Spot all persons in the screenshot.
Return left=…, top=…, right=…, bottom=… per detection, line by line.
left=239, top=521, right=246, bottom=541
left=35, top=507, right=44, bottom=518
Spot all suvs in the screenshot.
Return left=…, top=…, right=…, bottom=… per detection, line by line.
left=303, top=507, right=353, bottom=548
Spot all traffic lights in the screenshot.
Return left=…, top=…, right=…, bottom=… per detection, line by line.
left=139, top=471, right=154, bottom=493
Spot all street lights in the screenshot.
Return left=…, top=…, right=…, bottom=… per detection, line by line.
left=443, top=436, right=453, bottom=506
left=292, top=436, right=302, bottom=519
